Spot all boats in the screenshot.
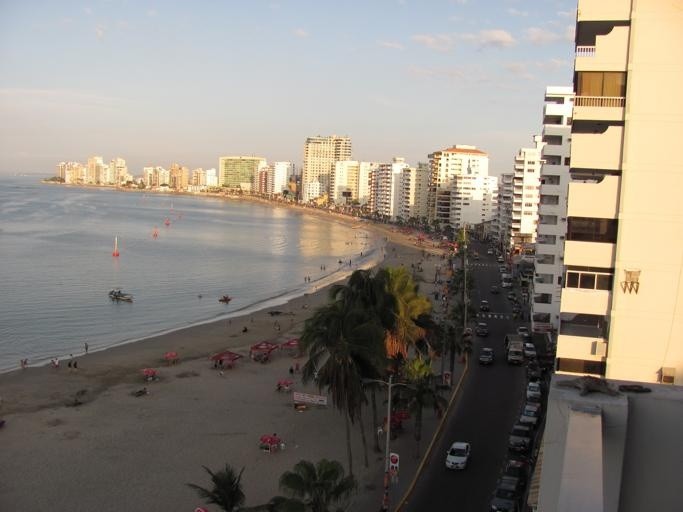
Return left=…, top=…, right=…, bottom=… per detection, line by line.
left=107, top=286, right=133, bottom=303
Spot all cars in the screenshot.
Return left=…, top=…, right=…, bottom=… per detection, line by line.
left=445, top=441, right=471, bottom=471
left=479, top=299, right=489, bottom=312
left=487, top=241, right=542, bottom=512
left=474, top=323, right=488, bottom=337
left=478, top=347, right=495, bottom=367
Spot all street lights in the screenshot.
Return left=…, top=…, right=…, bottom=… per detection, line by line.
left=366, top=374, right=419, bottom=511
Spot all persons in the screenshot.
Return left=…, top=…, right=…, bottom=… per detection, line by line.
left=132, top=317, right=316, bottom=398
left=268, top=432, right=280, bottom=455
left=399, top=220, right=459, bottom=324
left=19, top=341, right=89, bottom=371
